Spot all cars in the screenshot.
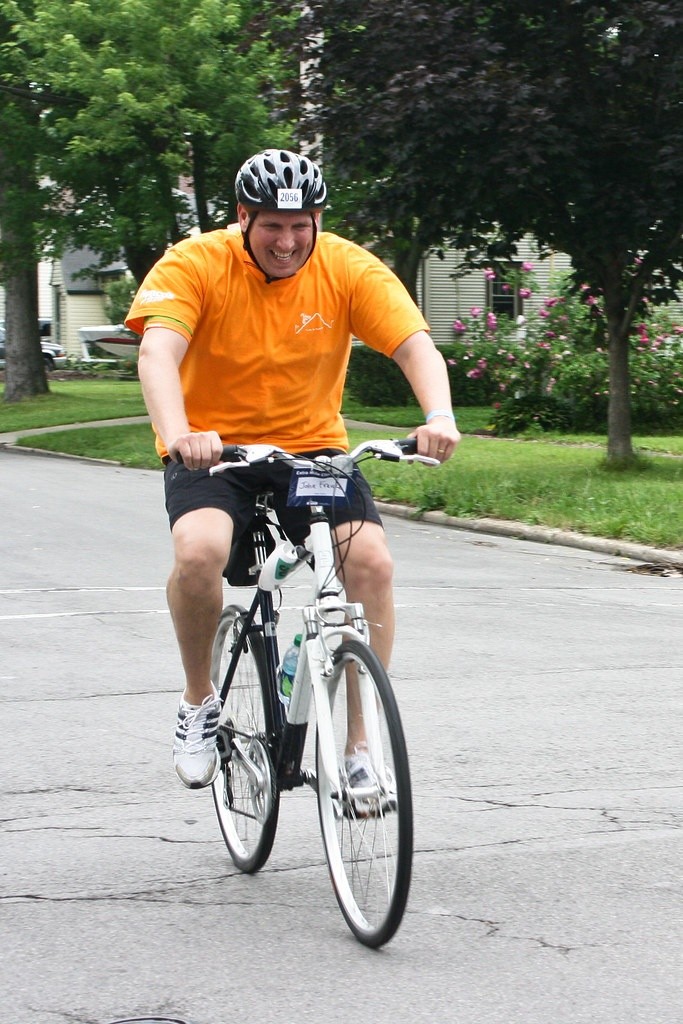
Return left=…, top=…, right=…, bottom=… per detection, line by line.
left=0, top=328, right=66, bottom=372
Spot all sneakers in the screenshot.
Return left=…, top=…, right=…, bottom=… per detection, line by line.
left=341, top=761, right=396, bottom=814
left=172, top=680, right=221, bottom=789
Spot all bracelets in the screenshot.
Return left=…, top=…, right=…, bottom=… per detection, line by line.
left=424, top=408, right=456, bottom=424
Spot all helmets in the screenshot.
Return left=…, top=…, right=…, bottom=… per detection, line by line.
left=234, top=148, right=329, bottom=212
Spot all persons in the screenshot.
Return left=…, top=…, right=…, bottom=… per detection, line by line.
left=127, top=145, right=463, bottom=822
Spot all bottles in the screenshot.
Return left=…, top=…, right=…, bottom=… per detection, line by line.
left=277, top=633, right=304, bottom=705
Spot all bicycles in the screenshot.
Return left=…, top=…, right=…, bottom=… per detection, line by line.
left=174, top=436, right=443, bottom=949
